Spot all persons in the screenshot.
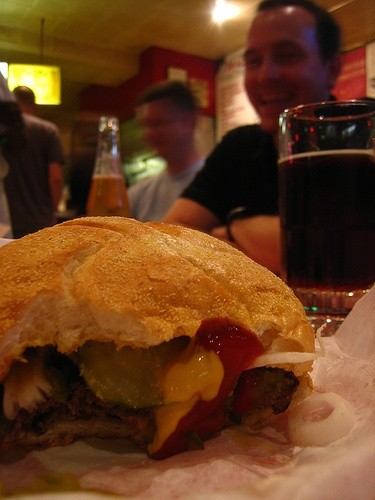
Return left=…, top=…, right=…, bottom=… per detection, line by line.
left=0, top=71, right=208, bottom=238
left=162, top=0, right=346, bottom=277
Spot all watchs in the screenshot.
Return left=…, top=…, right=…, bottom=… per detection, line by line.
left=225, top=206, right=253, bottom=247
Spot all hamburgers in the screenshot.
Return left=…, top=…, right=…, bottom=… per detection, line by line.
left=0, top=215, right=351, bottom=461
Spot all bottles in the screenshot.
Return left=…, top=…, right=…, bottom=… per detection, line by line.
left=87, top=115, right=134, bottom=221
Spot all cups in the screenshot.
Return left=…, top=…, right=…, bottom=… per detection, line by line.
left=278, top=100, right=375, bottom=315
